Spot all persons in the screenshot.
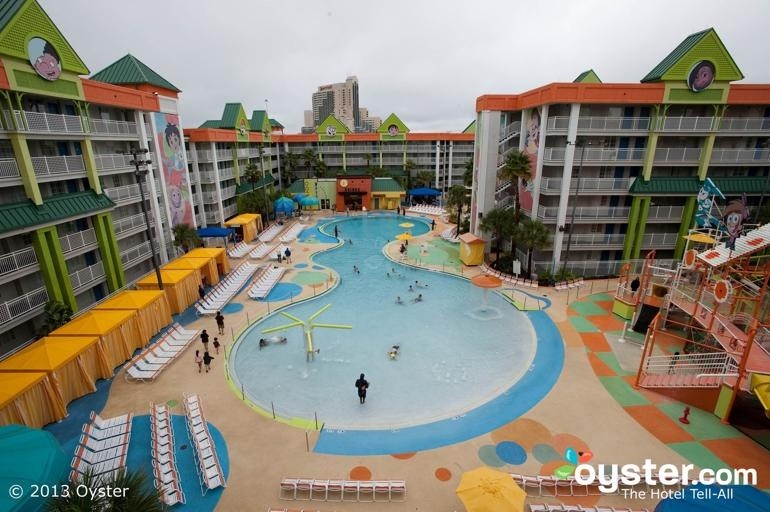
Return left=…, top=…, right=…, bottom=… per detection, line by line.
left=388, top=343, right=401, bottom=361
left=202, top=351, right=215, bottom=373
left=415, top=280, right=432, bottom=288
left=402, top=207, right=405, bottom=216
left=357, top=270, right=360, bottom=275
left=284, top=247, right=293, bottom=264
left=355, top=373, right=370, bottom=406
left=213, top=337, right=221, bottom=355
left=665, top=352, right=680, bottom=375
left=215, top=311, right=225, bottom=336
left=396, top=205, right=400, bottom=215
left=200, top=330, right=210, bottom=351
left=408, top=285, right=414, bottom=290
left=194, top=350, right=203, bottom=373
left=349, top=240, right=353, bottom=244
left=630, top=276, right=641, bottom=298
left=352, top=264, right=358, bottom=272
left=400, top=243, right=406, bottom=263
left=385, top=272, right=390, bottom=276
left=259, top=336, right=287, bottom=352
left=414, top=294, right=423, bottom=302
left=334, top=225, right=338, bottom=238
left=390, top=267, right=396, bottom=273
left=430, top=219, right=437, bottom=230
left=276, top=250, right=281, bottom=263
left=395, top=296, right=403, bottom=304
left=404, top=239, right=408, bottom=257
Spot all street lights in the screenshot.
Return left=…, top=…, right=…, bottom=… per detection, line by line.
left=257, top=142, right=271, bottom=226
left=436, top=143, right=453, bottom=204
left=560, top=136, right=609, bottom=282
left=128, top=145, right=165, bottom=296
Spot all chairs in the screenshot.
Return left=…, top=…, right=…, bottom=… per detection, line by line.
left=68, top=221, right=310, bottom=512
left=510, top=472, right=694, bottom=512
left=406, top=203, right=584, bottom=293
left=311, top=478, right=408, bottom=504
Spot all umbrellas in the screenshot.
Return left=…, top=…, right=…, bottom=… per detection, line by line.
left=274, top=198, right=295, bottom=216
left=292, top=193, right=307, bottom=202
left=299, top=196, right=320, bottom=208
left=273, top=196, right=294, bottom=206
